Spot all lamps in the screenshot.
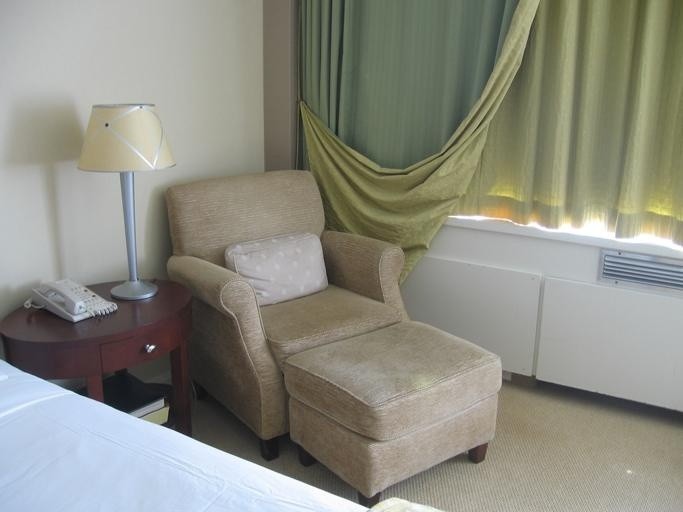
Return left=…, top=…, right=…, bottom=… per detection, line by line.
left=76, top=102, right=177, bottom=302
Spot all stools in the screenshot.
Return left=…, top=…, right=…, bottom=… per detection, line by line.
left=281, top=320, right=502, bottom=507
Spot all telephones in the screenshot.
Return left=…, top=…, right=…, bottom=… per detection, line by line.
left=23, top=276, right=118, bottom=324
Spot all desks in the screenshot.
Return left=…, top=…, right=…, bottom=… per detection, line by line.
left=0, top=278, right=197, bottom=436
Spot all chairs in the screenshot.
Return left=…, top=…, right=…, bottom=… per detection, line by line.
left=167, top=168, right=410, bottom=462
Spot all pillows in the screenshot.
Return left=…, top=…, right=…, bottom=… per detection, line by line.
left=223, top=231, right=330, bottom=307
